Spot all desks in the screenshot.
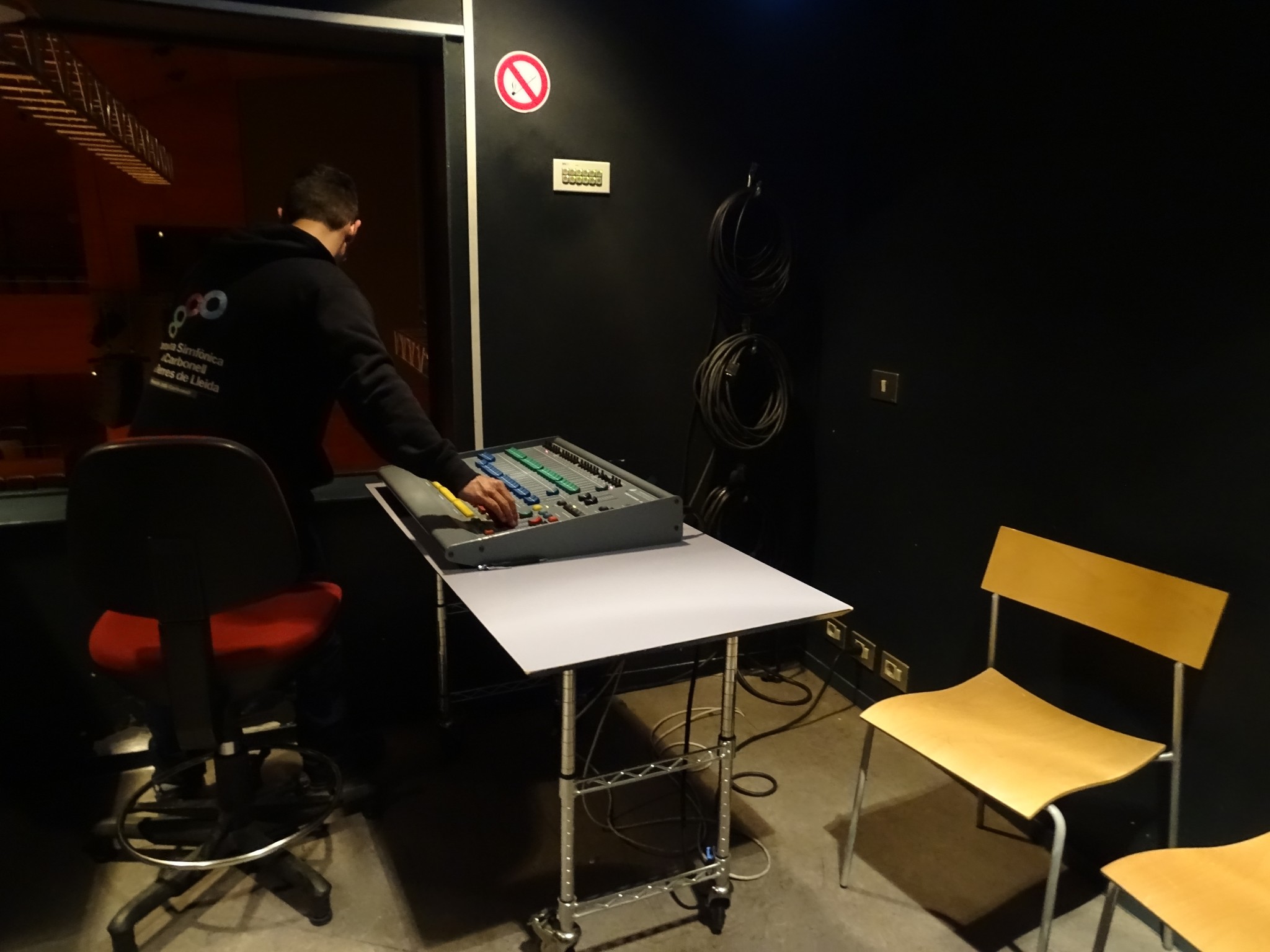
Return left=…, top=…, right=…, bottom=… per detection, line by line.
left=363, top=485, right=854, bottom=952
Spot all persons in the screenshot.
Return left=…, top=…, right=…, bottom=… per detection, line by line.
left=143, top=164, right=519, bottom=808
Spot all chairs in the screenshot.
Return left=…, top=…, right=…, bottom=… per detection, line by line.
left=75, top=441, right=389, bottom=952
left=1093, top=829, right=1270, bottom=952
left=839, top=525, right=1229, bottom=952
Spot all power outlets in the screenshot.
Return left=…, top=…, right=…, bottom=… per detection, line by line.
left=849, top=631, right=875, bottom=671
left=825, top=616, right=847, bottom=650
left=880, top=651, right=909, bottom=694
